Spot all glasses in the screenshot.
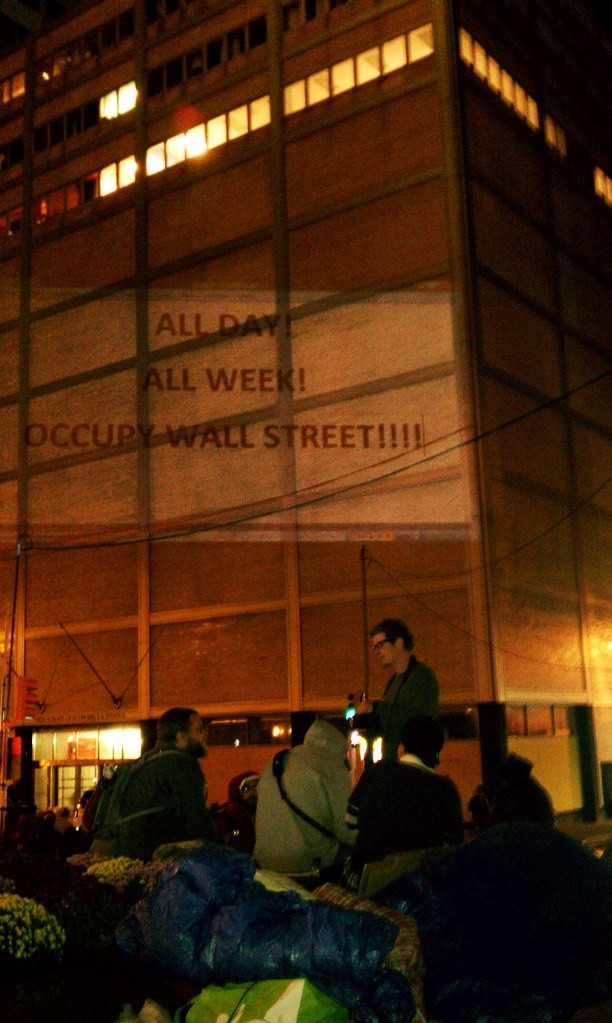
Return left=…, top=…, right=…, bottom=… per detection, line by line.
left=240, top=783, right=257, bottom=793
left=371, top=637, right=395, bottom=652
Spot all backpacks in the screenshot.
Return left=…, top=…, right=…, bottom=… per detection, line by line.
left=91, top=748, right=190, bottom=841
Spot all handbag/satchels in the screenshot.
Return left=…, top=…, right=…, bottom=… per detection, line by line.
left=174, top=977, right=349, bottom=1023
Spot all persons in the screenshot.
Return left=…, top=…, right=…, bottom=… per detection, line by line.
left=35, top=789, right=94, bottom=851
left=350, top=618, right=440, bottom=764
left=344, top=714, right=464, bottom=896
left=468, top=752, right=597, bottom=863
left=253, top=720, right=351, bottom=892
left=92, top=706, right=211, bottom=861
left=214, top=771, right=261, bottom=834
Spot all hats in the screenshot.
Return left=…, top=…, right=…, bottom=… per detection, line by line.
left=400, top=714, right=445, bottom=767
left=239, top=775, right=260, bottom=791
